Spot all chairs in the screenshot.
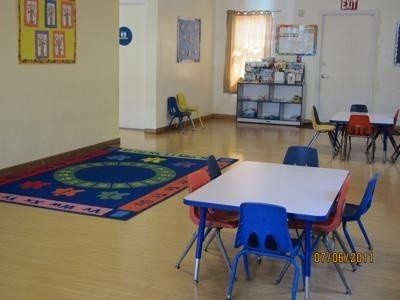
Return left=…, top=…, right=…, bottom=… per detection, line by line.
left=175, top=146, right=381, bottom=300
left=167, top=94, right=205, bottom=131
left=308, top=103, right=400, bottom=164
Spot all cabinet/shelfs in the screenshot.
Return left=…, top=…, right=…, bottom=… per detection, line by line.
left=236, top=66, right=306, bottom=125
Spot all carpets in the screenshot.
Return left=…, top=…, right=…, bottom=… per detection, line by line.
left=1, top=146, right=239, bottom=221
